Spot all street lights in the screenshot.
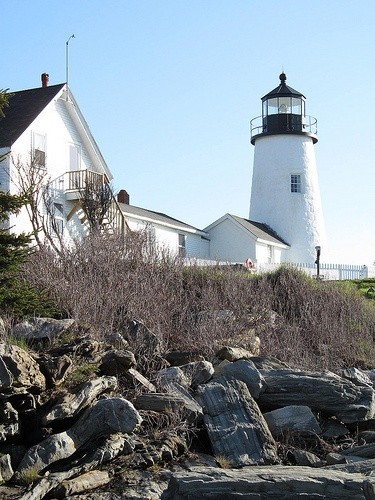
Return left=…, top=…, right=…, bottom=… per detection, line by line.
left=314, top=246, right=321, bottom=281
left=64, top=34, right=75, bottom=100
